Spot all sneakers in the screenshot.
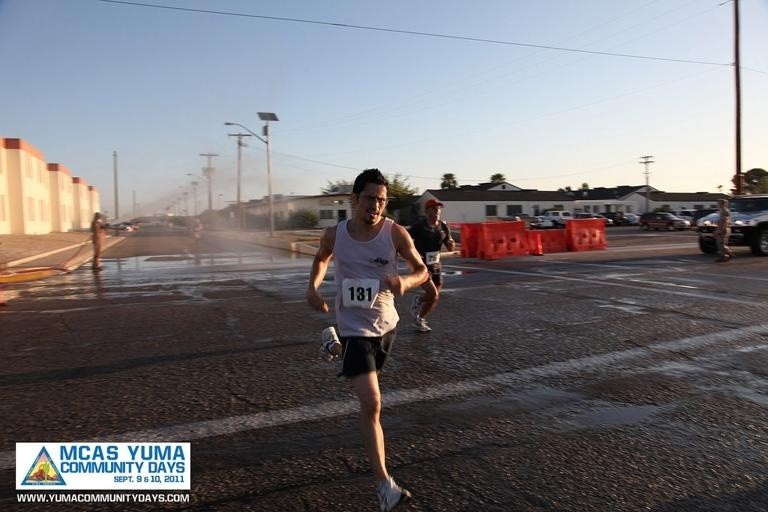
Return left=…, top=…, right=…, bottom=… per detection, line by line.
left=415, top=315, right=432, bottom=332
left=376, top=476, right=412, bottom=512
left=410, top=294, right=423, bottom=318
left=320, top=328, right=341, bottom=362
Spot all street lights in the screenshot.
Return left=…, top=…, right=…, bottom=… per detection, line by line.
left=187, top=172, right=214, bottom=230
left=155, top=184, right=199, bottom=227
left=224, top=123, right=275, bottom=238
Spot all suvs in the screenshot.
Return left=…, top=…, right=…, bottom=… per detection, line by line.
left=696, top=194, right=768, bottom=257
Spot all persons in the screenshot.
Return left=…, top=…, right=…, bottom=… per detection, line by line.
left=91, top=212, right=103, bottom=269
left=716, top=200, right=737, bottom=261
left=306, top=168, right=429, bottom=512
left=408, top=199, right=456, bottom=332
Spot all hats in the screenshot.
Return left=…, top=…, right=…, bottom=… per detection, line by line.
left=425, top=199, right=443, bottom=208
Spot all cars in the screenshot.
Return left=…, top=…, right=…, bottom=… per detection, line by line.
left=511, top=208, right=720, bottom=231
left=109, top=213, right=193, bottom=236
